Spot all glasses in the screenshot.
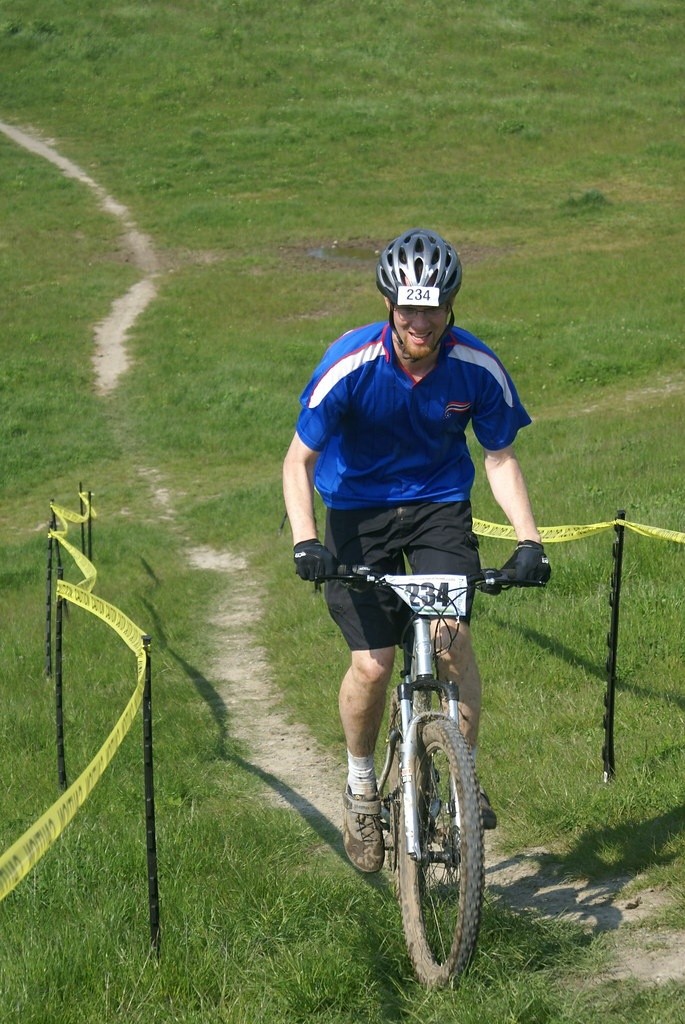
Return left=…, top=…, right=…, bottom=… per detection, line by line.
left=390, top=306, right=446, bottom=321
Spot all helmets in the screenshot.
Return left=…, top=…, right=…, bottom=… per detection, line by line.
left=376, top=227, right=462, bottom=304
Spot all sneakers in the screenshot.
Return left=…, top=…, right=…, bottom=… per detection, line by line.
left=480, top=784, right=496, bottom=829
left=343, top=784, right=384, bottom=872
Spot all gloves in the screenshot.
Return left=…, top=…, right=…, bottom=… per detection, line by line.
left=501, top=540, right=551, bottom=586
left=293, top=538, right=341, bottom=582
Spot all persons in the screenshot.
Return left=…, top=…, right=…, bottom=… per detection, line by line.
left=281, top=228, right=552, bottom=871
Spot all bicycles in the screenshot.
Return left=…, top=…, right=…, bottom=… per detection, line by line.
left=293, top=562, right=547, bottom=995
